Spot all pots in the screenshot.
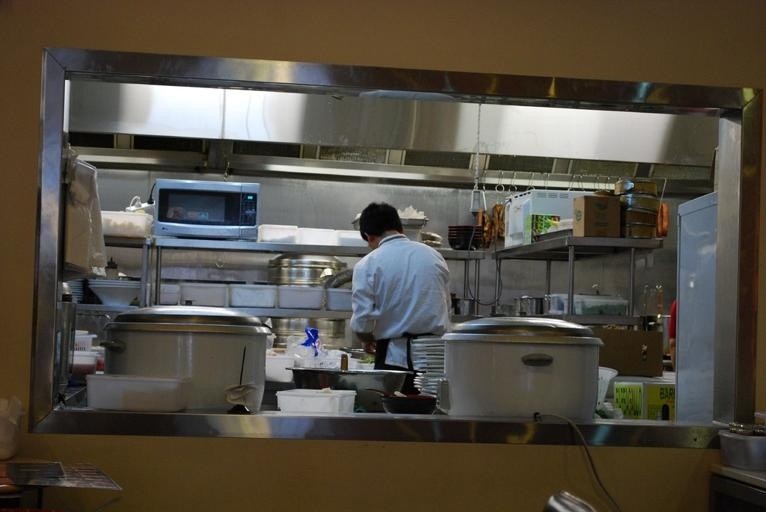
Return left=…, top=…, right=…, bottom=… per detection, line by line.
left=451, top=297, right=479, bottom=316
left=490, top=303, right=516, bottom=317
left=513, top=296, right=544, bottom=316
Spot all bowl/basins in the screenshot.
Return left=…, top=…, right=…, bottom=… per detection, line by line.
left=379, top=395, right=436, bottom=415
left=447, top=224, right=481, bottom=250
left=285, top=363, right=415, bottom=414
left=87, top=278, right=144, bottom=306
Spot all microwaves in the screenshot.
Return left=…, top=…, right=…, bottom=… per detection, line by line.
left=148, top=179, right=259, bottom=241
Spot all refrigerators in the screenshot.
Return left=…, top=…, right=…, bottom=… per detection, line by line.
left=674, top=190, right=715, bottom=423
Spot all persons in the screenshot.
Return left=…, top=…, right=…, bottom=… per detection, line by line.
left=348, top=201, right=450, bottom=398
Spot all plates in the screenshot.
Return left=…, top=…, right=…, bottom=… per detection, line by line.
left=61, top=278, right=84, bottom=303
left=410, top=337, right=445, bottom=399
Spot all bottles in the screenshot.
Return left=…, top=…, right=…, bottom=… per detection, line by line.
left=104, top=257, right=119, bottom=280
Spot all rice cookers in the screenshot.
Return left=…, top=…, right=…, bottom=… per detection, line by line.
left=435, top=313, right=606, bottom=426
left=99, top=301, right=274, bottom=413
